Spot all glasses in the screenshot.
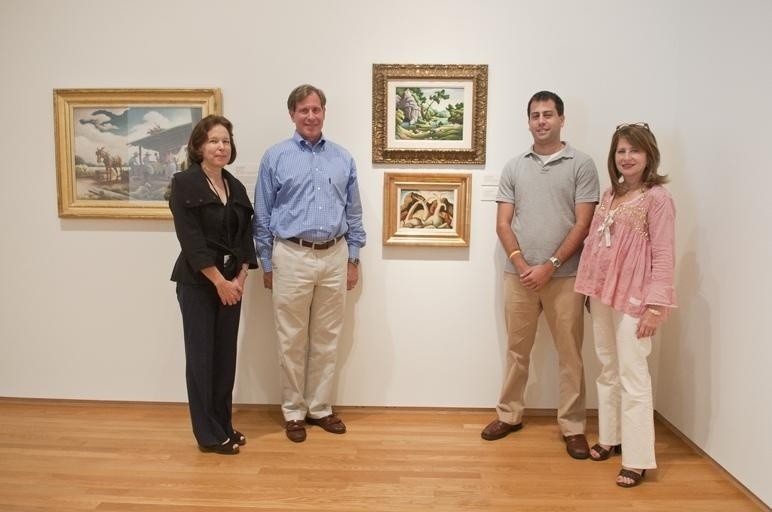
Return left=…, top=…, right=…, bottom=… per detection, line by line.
left=616, top=122, right=648, bottom=130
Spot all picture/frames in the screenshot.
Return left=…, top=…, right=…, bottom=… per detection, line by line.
left=371, top=62, right=487, bottom=167
left=383, top=172, right=472, bottom=248
left=53, top=87, right=222, bottom=220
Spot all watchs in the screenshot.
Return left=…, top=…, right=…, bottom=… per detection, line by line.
left=348, top=258, right=360, bottom=267
left=549, top=256, right=562, bottom=270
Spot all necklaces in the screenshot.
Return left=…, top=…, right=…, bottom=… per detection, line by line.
left=205, top=173, right=229, bottom=205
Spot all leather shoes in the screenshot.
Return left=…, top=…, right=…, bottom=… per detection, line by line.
left=482, top=421, right=522, bottom=441
left=305, top=413, right=347, bottom=433
left=564, top=433, right=590, bottom=459
left=286, top=422, right=306, bottom=442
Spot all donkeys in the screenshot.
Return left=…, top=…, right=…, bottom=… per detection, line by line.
left=96, top=147, right=123, bottom=182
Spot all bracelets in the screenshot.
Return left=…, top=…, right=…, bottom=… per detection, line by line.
left=241, top=265, right=249, bottom=281
left=646, top=307, right=663, bottom=317
left=509, top=248, right=522, bottom=260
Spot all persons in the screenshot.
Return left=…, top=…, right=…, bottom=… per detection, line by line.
left=167, top=114, right=259, bottom=457
left=128, top=152, right=159, bottom=176
left=481, top=91, right=601, bottom=460
left=573, top=125, right=676, bottom=488
left=252, top=83, right=366, bottom=444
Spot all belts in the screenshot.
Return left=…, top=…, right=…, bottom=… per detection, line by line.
left=288, top=236, right=343, bottom=250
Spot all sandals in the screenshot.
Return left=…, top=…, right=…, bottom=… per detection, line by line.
left=200, top=429, right=245, bottom=456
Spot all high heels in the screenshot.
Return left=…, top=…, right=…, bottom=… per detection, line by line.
left=617, top=470, right=645, bottom=487
left=592, top=444, right=621, bottom=461
